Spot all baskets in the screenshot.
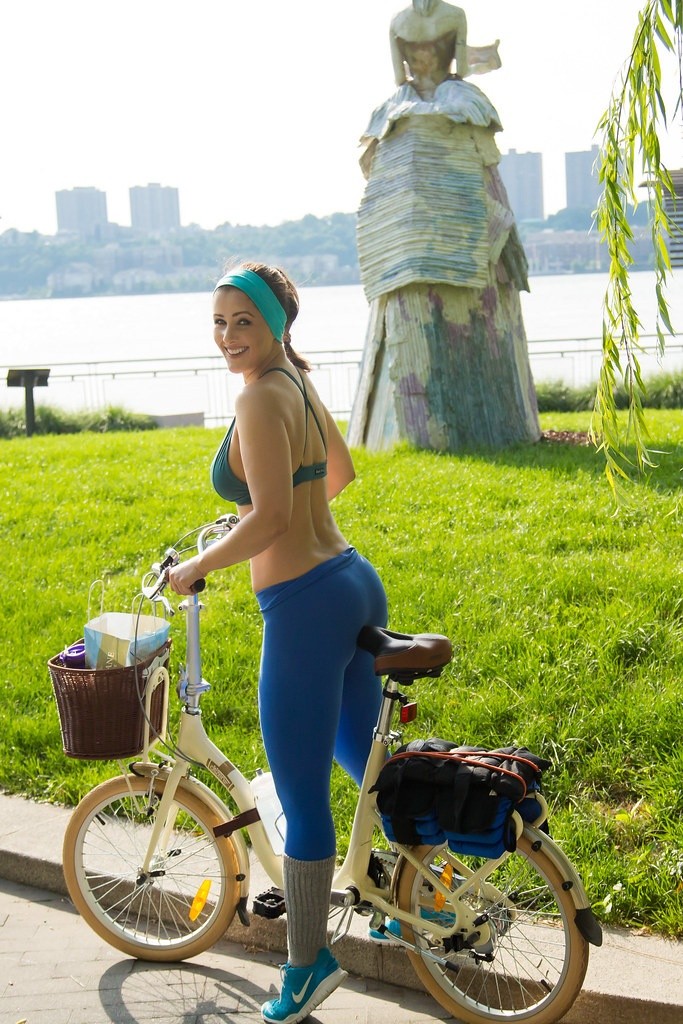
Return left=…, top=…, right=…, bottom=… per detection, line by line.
left=47, top=636, right=174, bottom=760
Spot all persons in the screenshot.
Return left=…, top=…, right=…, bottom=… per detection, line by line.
left=389, top=1, right=491, bottom=103
left=165, top=262, right=460, bottom=1024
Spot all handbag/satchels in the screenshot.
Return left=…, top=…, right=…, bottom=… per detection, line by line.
left=83, top=579, right=172, bottom=670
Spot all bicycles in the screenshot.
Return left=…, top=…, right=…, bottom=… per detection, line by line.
left=46, top=513, right=602, bottom=1024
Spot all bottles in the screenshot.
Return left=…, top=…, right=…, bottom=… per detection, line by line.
left=250, top=767, right=288, bottom=856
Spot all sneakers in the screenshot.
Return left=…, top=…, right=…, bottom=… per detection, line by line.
left=368, top=897, right=458, bottom=944
left=261, top=946, right=350, bottom=1024
